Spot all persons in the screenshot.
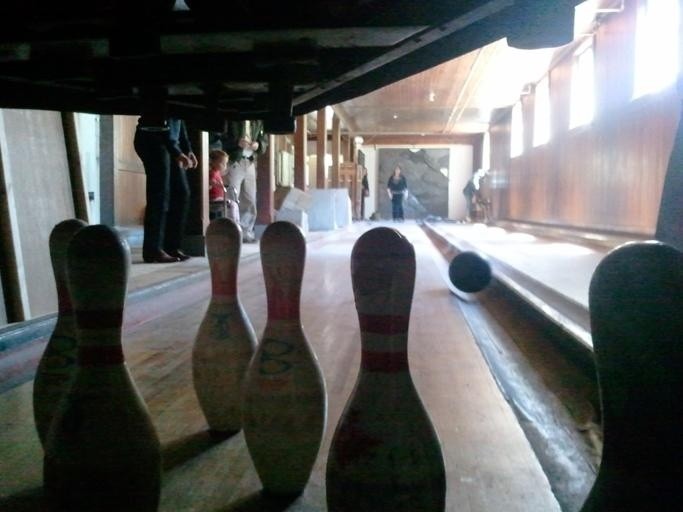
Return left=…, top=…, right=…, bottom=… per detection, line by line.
left=386, top=165, right=408, bottom=221
left=227, top=119, right=270, bottom=245
left=208, top=149, right=227, bottom=223
left=132, top=115, right=198, bottom=261
left=462, top=172, right=478, bottom=222
left=361, top=167, right=372, bottom=217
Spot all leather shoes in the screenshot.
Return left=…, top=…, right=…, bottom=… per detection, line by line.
left=143, top=249, right=189, bottom=263
